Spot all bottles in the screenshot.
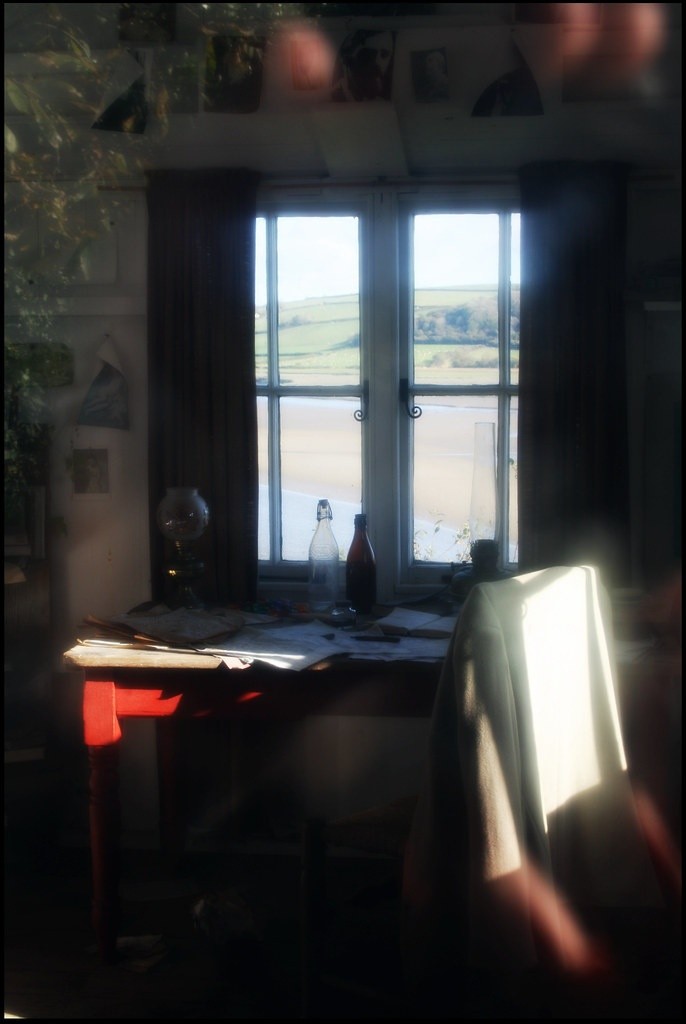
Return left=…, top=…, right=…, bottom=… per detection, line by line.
left=310, top=498, right=341, bottom=613
left=346, top=514, right=377, bottom=615
left=450, top=538, right=508, bottom=607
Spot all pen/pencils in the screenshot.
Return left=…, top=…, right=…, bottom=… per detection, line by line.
left=350, top=636, right=392, bottom=642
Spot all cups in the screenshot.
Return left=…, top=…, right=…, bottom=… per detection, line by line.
left=610, top=589, right=649, bottom=642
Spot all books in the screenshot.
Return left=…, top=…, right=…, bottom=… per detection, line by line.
left=374, top=607, right=456, bottom=638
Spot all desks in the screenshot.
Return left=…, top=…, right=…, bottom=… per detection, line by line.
left=61, top=609, right=458, bottom=962
